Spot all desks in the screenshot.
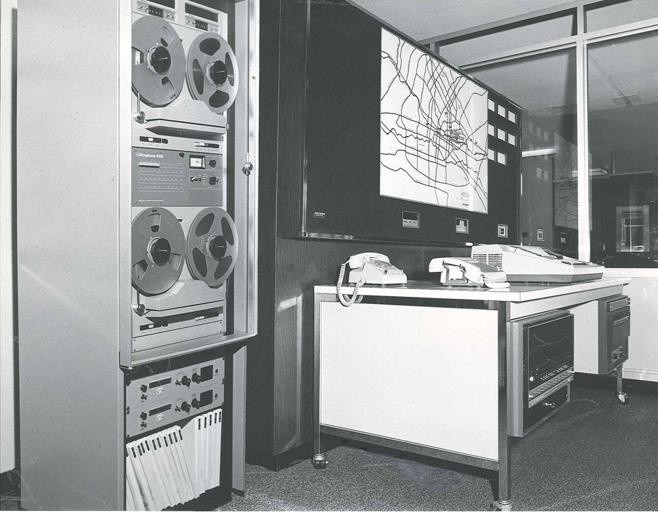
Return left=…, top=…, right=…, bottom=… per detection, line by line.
left=310, top=275, right=633, bottom=510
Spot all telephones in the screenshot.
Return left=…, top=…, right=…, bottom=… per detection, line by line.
left=348, top=252, right=408, bottom=285
left=429, top=256, right=506, bottom=287
